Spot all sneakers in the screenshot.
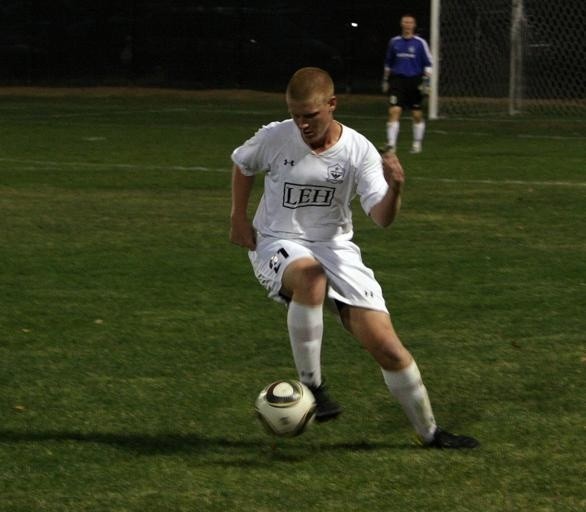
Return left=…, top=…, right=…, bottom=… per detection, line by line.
left=306, top=375, right=343, bottom=422
left=384, top=146, right=397, bottom=154
left=422, top=426, right=477, bottom=450
left=410, top=142, right=423, bottom=155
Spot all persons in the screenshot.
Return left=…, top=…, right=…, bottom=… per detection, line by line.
left=227, top=63, right=482, bottom=452
left=377, top=15, right=435, bottom=157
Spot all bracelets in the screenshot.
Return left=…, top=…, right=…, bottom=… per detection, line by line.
left=382, top=75, right=389, bottom=83
left=422, top=76, right=431, bottom=88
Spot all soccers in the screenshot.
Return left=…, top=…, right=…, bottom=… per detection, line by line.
left=255, top=379, right=317, bottom=438
